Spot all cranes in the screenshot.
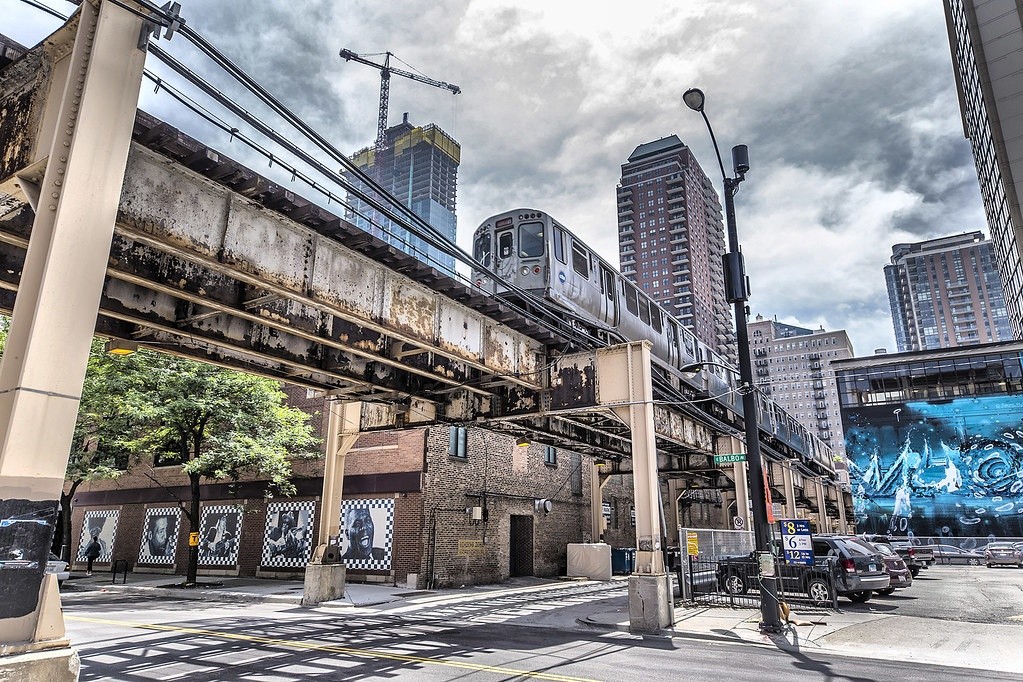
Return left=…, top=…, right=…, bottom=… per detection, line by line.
left=339, top=48, right=462, bottom=238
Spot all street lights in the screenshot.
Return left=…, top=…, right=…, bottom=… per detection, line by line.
left=682, top=88, right=782, bottom=634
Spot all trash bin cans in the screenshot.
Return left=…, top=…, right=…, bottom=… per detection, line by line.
left=611, top=547, right=636, bottom=575
left=666, top=545, right=687, bottom=573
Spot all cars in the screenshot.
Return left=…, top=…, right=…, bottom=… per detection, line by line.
left=45, top=553, right=71, bottom=587
left=715, top=535, right=891, bottom=607
left=927, top=545, right=986, bottom=566
left=967, top=542, right=1023, bottom=569
left=856, top=534, right=935, bottom=596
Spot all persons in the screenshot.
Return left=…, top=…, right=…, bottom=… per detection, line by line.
left=342, top=509, right=384, bottom=559
left=81, top=518, right=107, bottom=556
left=269, top=511, right=297, bottom=558
left=85, top=536, right=101, bottom=576
left=144, top=515, right=172, bottom=556
left=202, top=513, right=232, bottom=557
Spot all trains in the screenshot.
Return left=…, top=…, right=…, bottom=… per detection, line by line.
left=471, top=208, right=836, bottom=482
